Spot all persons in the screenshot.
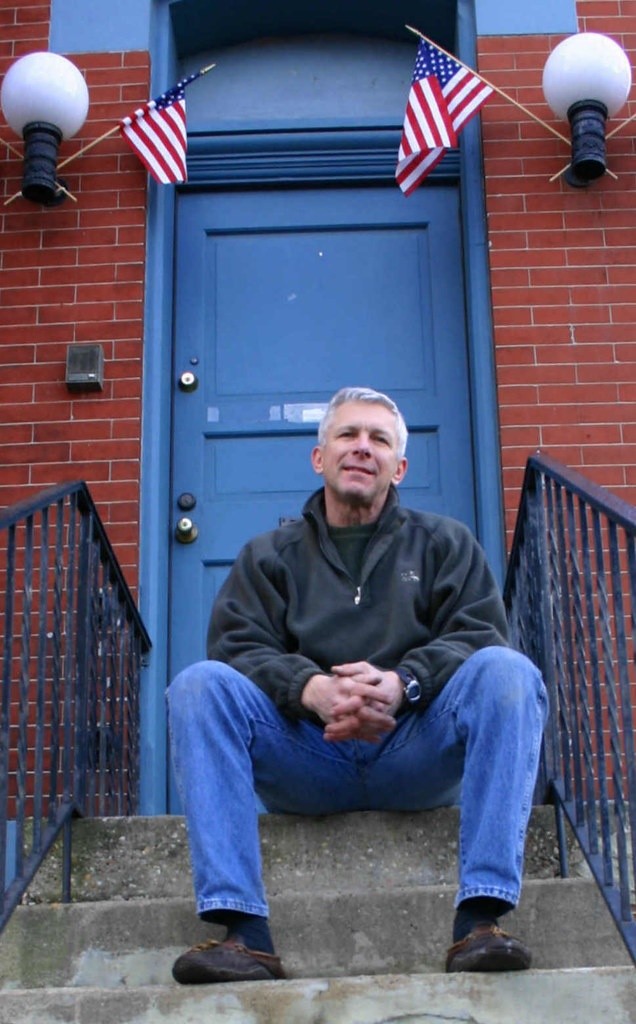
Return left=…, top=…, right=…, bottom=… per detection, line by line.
left=168, top=384, right=549, bottom=983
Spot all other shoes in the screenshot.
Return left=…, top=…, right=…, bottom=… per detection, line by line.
left=172, top=935, right=284, bottom=984
left=446, top=924, right=533, bottom=972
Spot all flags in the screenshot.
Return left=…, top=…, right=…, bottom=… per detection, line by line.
left=394, top=38, right=496, bottom=199
left=117, top=67, right=202, bottom=184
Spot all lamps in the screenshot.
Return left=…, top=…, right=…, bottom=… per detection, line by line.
left=542, top=31, right=632, bottom=187
left=0, top=50, right=89, bottom=206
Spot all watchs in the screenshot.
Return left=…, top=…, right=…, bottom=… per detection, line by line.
left=392, top=666, right=421, bottom=707
